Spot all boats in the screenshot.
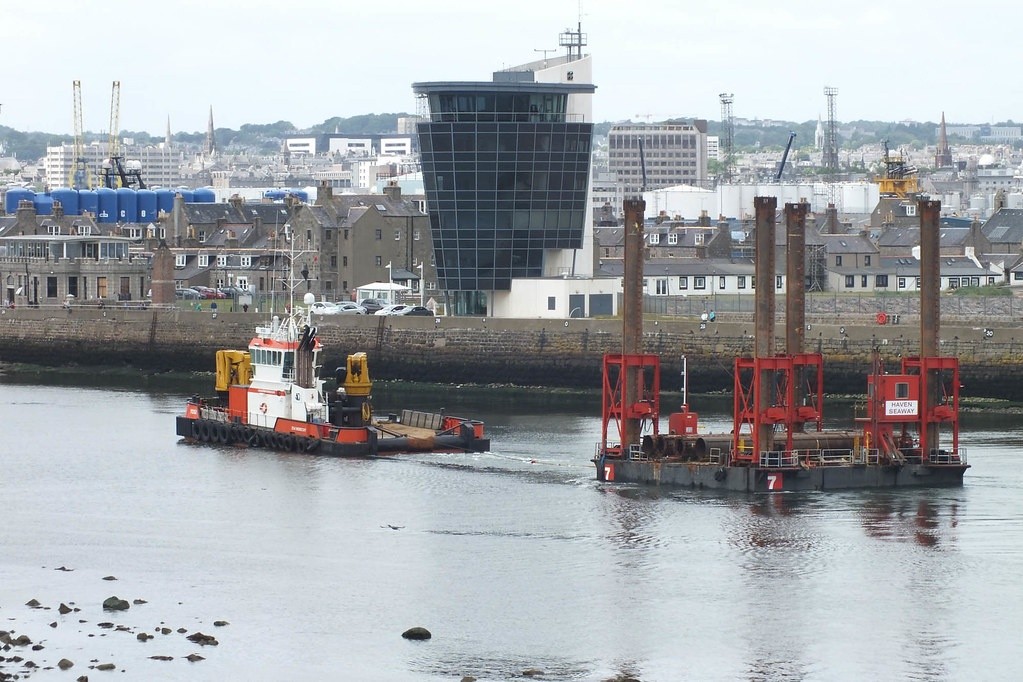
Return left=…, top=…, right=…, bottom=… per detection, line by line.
left=176, top=233, right=491, bottom=458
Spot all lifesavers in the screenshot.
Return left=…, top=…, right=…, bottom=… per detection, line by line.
left=201, top=424, right=210, bottom=442
left=270, top=433, right=279, bottom=448
left=878, top=315, right=886, bottom=324
left=276, top=435, right=285, bottom=449
left=230, top=426, right=238, bottom=442
left=260, top=403, right=268, bottom=413
left=192, top=421, right=202, bottom=439
left=242, top=430, right=251, bottom=442
left=210, top=424, right=219, bottom=441
left=284, top=437, right=294, bottom=452
left=263, top=433, right=271, bottom=447
left=219, top=426, right=229, bottom=444
left=236, top=429, right=243, bottom=441
left=249, top=434, right=260, bottom=447
left=297, top=438, right=307, bottom=453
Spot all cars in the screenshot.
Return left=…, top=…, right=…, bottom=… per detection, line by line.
left=175, top=285, right=252, bottom=300
left=310, top=298, right=434, bottom=316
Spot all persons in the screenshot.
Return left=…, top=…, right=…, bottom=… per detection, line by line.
left=701, top=310, right=708, bottom=322
left=710, top=310, right=715, bottom=322
left=211, top=300, right=217, bottom=312
left=62, top=300, right=70, bottom=308
left=284, top=304, right=290, bottom=313
left=243, top=303, right=248, bottom=312
left=194, top=302, right=202, bottom=311
left=98, top=299, right=104, bottom=309
left=7, top=300, right=15, bottom=309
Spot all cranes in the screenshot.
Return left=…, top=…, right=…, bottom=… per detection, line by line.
left=99, top=81, right=123, bottom=188
left=69, top=80, right=92, bottom=190
